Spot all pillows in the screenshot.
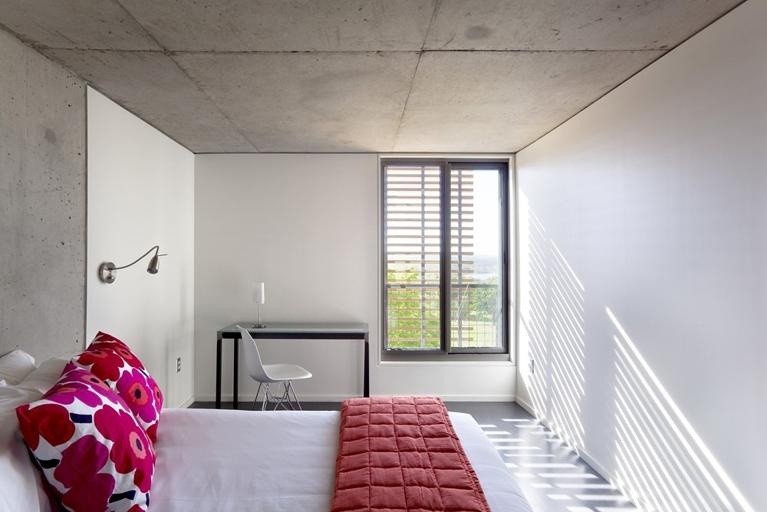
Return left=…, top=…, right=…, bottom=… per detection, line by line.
left=75, top=330, right=163, bottom=444
left=0, top=380, right=53, bottom=512
left=16, top=357, right=157, bottom=512
left=17, top=354, right=74, bottom=392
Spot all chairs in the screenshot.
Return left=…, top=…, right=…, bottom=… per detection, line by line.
left=236, top=324, right=313, bottom=411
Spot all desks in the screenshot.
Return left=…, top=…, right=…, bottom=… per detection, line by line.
left=215, top=321, right=370, bottom=408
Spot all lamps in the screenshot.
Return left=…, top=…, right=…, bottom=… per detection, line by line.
left=250, top=281, right=266, bottom=329
left=98, top=246, right=167, bottom=284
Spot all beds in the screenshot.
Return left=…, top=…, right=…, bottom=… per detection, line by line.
left=0, top=348, right=536, bottom=512
